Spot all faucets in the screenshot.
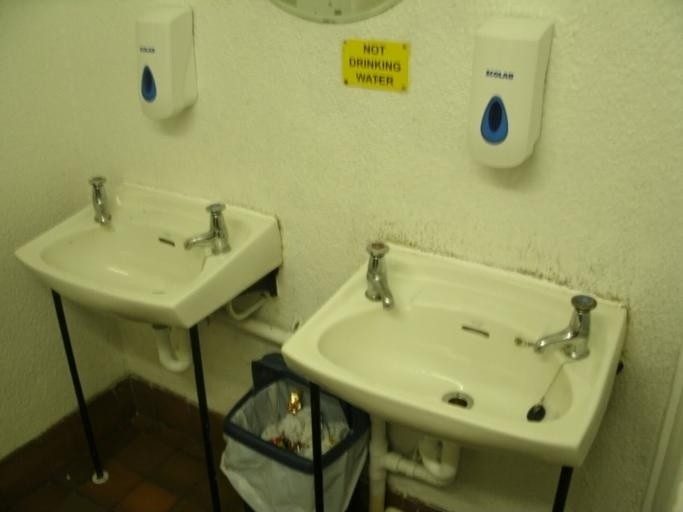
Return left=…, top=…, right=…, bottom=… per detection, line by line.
left=363, top=240, right=396, bottom=309
left=182, top=202, right=231, bottom=256
left=89, top=174, right=113, bottom=225
left=533, top=293, right=599, bottom=360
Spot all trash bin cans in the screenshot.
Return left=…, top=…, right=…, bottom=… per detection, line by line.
left=222, top=376, right=371, bottom=512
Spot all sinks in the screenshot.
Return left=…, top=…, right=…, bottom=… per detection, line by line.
left=280, top=242, right=632, bottom=469
left=12, top=177, right=285, bottom=328
